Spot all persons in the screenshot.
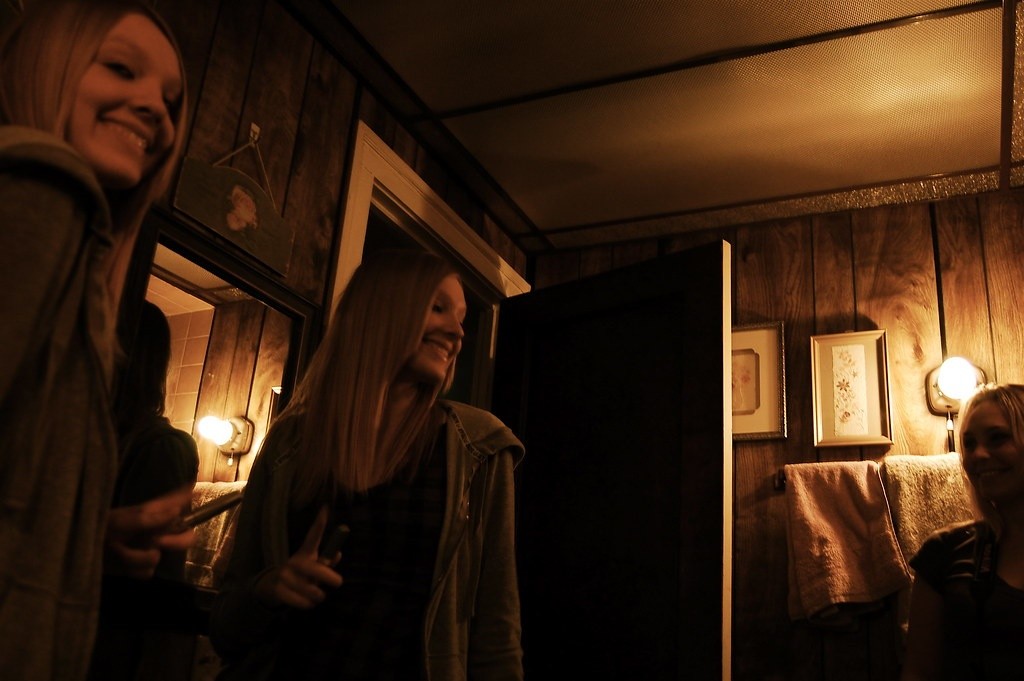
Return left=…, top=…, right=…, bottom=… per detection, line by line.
left=0, top=0, right=211, bottom=681
left=899, top=385, right=1024, bottom=681
left=230, top=248, right=524, bottom=681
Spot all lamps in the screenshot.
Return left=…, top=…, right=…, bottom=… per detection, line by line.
left=925, top=354, right=987, bottom=431
left=195, top=412, right=253, bottom=466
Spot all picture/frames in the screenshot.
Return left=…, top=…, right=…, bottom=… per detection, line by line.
left=731, top=319, right=788, bottom=440
left=810, top=328, right=894, bottom=447
